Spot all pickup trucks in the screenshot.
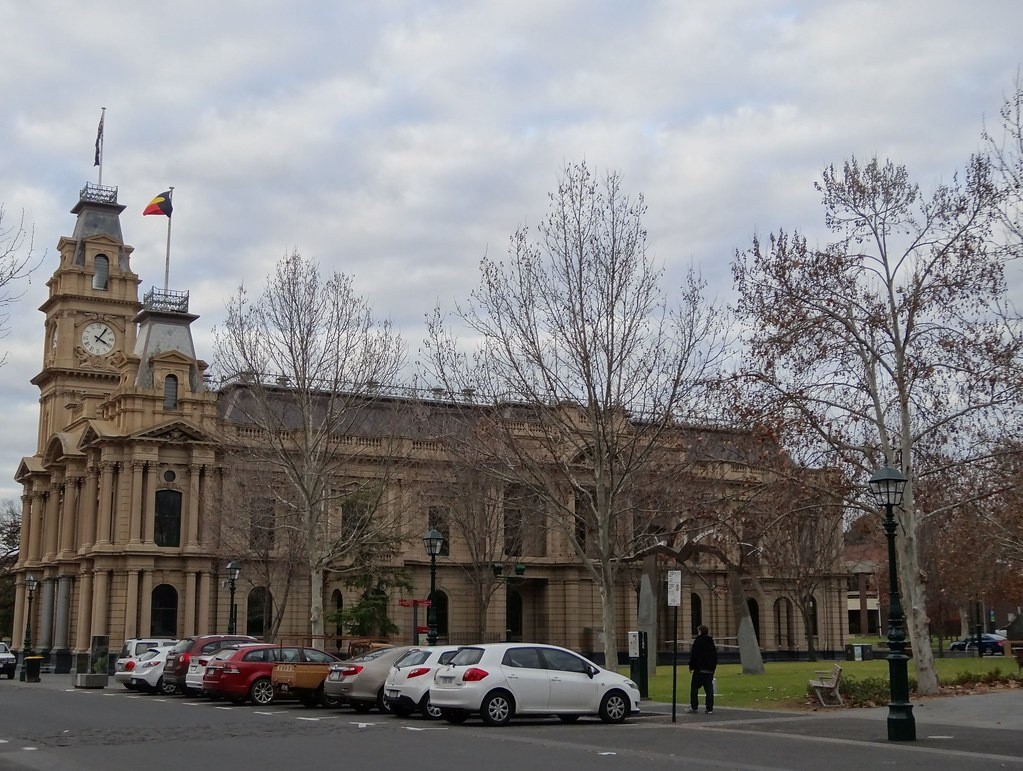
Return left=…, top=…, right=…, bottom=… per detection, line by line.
left=270, top=641, right=394, bottom=709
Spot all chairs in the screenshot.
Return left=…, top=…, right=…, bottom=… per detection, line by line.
left=291, top=652, right=299, bottom=662
left=283, top=653, right=289, bottom=663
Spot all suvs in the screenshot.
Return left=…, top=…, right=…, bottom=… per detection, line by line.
left=163, top=636, right=267, bottom=698
left=114, top=636, right=182, bottom=691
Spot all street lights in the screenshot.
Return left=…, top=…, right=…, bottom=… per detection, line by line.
left=225, top=561, right=242, bottom=634
left=868, top=467, right=918, bottom=742
left=22, top=574, right=40, bottom=652
left=423, top=523, right=445, bottom=645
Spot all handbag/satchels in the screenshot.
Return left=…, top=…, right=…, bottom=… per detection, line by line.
left=702, top=677, right=717, bottom=695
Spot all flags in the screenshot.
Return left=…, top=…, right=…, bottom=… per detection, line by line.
left=93, top=110, right=104, bottom=166
left=143, top=191, right=173, bottom=218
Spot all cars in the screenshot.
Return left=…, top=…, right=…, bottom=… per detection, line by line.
left=131, top=645, right=216, bottom=695
left=383, top=644, right=522, bottom=721
left=949, top=633, right=1007, bottom=656
left=202, top=644, right=343, bottom=706
left=184, top=643, right=268, bottom=701
left=323, top=645, right=426, bottom=713
left=0, top=642, right=16, bottom=680
left=429, top=641, right=641, bottom=727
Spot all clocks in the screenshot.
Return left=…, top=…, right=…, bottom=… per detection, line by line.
left=52, top=326, right=57, bottom=352
left=82, top=323, right=116, bottom=356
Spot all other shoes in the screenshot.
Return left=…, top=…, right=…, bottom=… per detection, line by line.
left=704, top=710, right=713, bottom=714
left=685, top=704, right=698, bottom=713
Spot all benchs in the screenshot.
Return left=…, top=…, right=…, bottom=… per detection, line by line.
left=808, top=663, right=843, bottom=708
left=876, top=641, right=911, bottom=649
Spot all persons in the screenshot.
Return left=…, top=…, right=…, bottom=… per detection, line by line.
left=685, top=624, right=719, bottom=714
